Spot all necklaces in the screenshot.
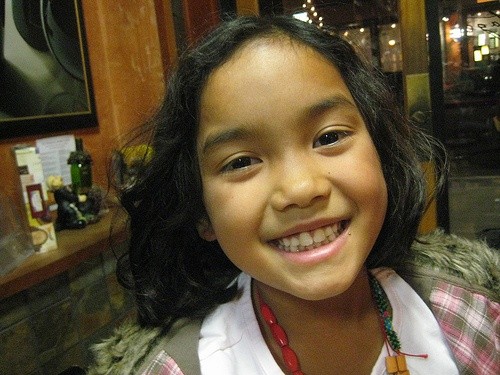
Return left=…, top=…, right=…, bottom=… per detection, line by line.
left=256, top=269, right=428, bottom=375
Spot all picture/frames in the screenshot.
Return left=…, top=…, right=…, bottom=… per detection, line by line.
left=1, top=1, right=100, bottom=140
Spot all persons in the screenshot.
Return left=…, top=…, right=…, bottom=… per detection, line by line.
left=87, top=13, right=500, bottom=375
left=46, top=174, right=87, bottom=233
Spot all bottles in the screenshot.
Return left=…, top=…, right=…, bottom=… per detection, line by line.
left=71, top=138, right=92, bottom=193
